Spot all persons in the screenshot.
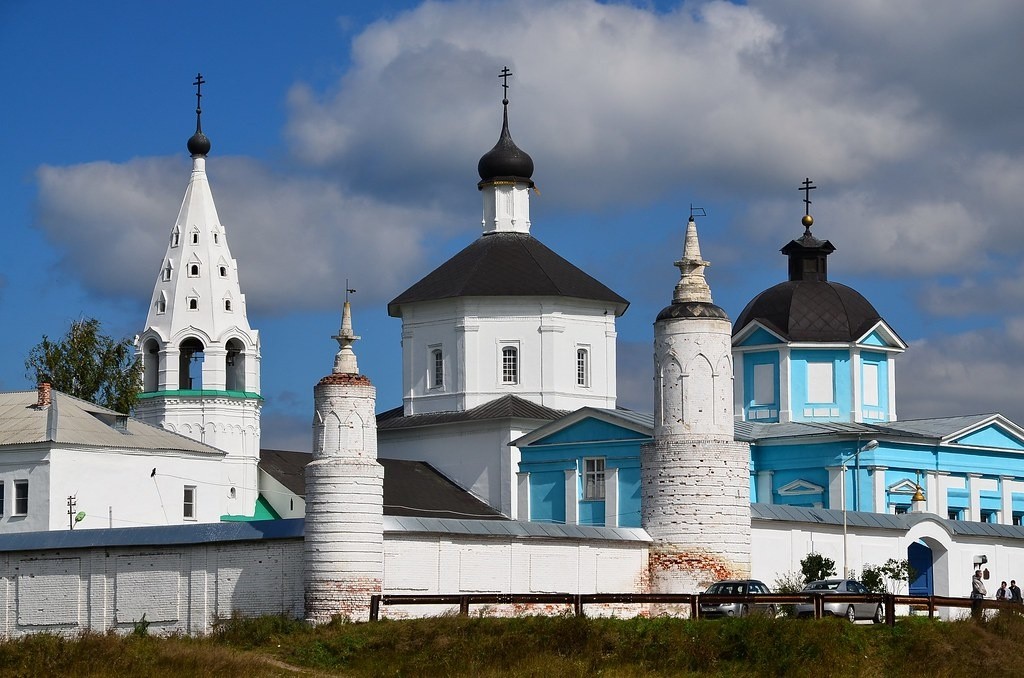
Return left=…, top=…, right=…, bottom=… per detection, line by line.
left=995, top=580, right=1023, bottom=615
left=970, top=570, right=986, bottom=619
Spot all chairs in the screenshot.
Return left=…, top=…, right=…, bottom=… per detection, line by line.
left=721, top=589, right=730, bottom=595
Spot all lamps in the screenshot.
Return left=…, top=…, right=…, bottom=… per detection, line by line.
left=984, top=568, right=990, bottom=580
left=73, top=511, right=85, bottom=530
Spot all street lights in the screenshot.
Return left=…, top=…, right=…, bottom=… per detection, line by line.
left=842, top=438, right=878, bottom=578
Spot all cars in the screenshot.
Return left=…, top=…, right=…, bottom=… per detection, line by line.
left=793, top=579, right=884, bottom=623
left=695, top=578, right=778, bottom=621
